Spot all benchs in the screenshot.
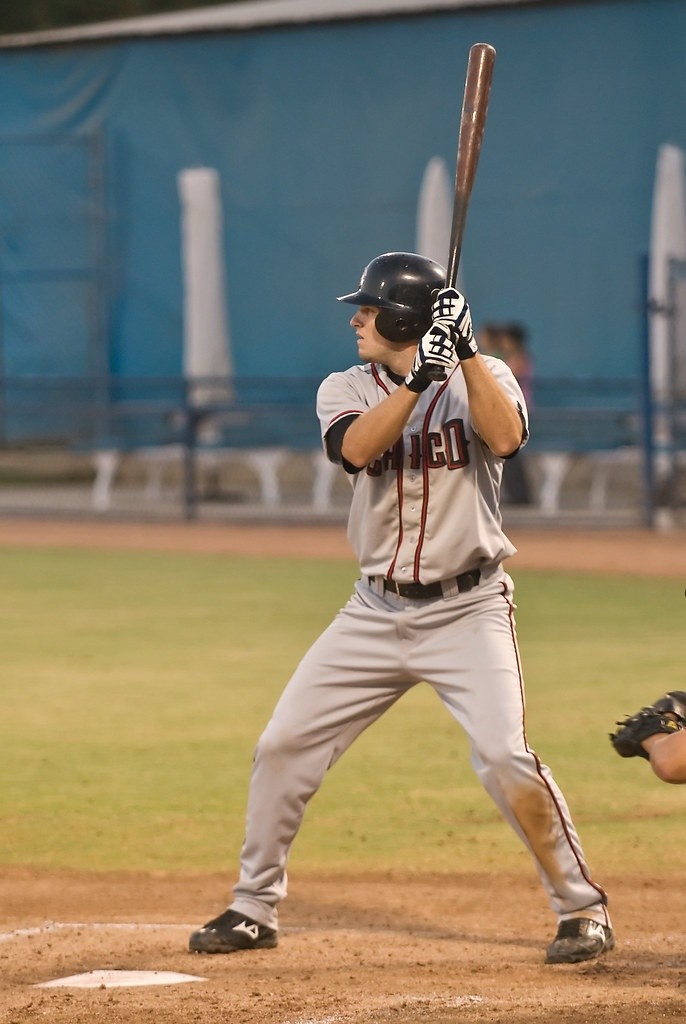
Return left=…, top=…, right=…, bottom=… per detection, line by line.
left=100, top=449, right=686, bottom=516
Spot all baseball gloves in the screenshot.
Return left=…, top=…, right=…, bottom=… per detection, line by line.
left=609, top=689, right=686, bottom=759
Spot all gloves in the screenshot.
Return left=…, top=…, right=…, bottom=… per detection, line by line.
left=405, top=287, right=479, bottom=395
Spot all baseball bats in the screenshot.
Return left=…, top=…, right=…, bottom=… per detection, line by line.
left=428, top=44, right=498, bottom=383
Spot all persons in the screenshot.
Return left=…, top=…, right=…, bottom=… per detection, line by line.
left=187, top=253, right=616, bottom=966
left=475, top=320, right=532, bottom=507
left=607, top=689, right=686, bottom=786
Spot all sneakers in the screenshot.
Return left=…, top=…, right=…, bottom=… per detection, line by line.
left=546, top=918, right=615, bottom=965
left=189, top=910, right=278, bottom=955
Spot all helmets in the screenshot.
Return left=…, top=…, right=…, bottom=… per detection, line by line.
left=336, top=252, right=447, bottom=342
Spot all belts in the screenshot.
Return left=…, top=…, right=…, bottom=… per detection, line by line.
left=368, top=569, right=481, bottom=602
